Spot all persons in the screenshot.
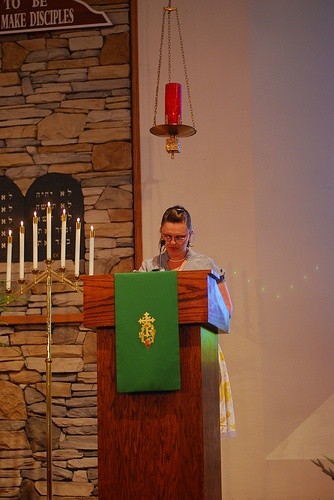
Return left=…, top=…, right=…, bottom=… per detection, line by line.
left=138, top=205, right=236, bottom=438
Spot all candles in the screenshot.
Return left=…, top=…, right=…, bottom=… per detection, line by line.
left=61, top=209, right=67, bottom=268
left=6, top=230, right=12, bottom=290
left=46, top=202, right=52, bottom=261
left=33, top=211, right=38, bottom=269
left=89, top=225, right=94, bottom=276
left=19, top=221, right=25, bottom=280
left=74, top=218, right=81, bottom=277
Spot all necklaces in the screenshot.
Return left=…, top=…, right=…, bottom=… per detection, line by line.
left=169, top=258, right=185, bottom=262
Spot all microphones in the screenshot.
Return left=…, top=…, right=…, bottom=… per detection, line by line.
left=152, top=240, right=165, bottom=271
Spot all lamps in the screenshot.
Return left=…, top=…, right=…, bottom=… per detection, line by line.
left=149, top=0, right=197, bottom=159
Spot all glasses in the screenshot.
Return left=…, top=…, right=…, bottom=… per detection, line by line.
left=162, top=232, right=190, bottom=241
